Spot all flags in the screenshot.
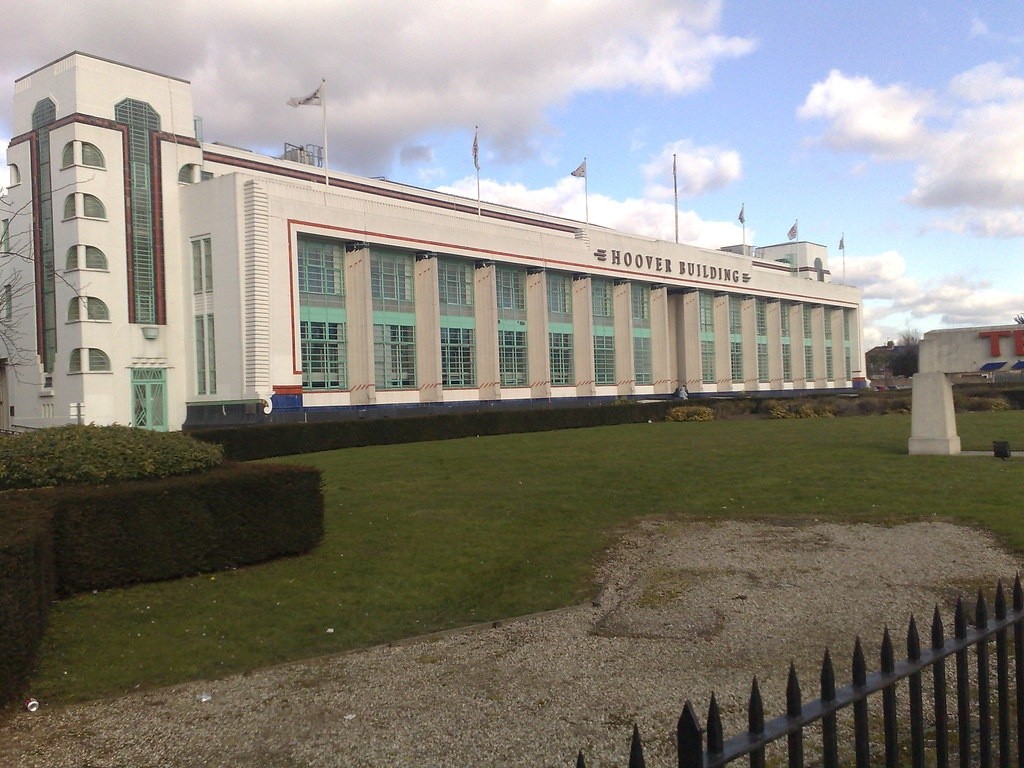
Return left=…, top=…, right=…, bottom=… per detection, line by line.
left=838, top=237, right=844, bottom=250
left=286, top=83, right=322, bottom=108
left=472, top=131, right=481, bottom=171
left=739, top=209, right=745, bottom=223
left=787, top=223, right=797, bottom=240
left=571, top=161, right=586, bottom=177
